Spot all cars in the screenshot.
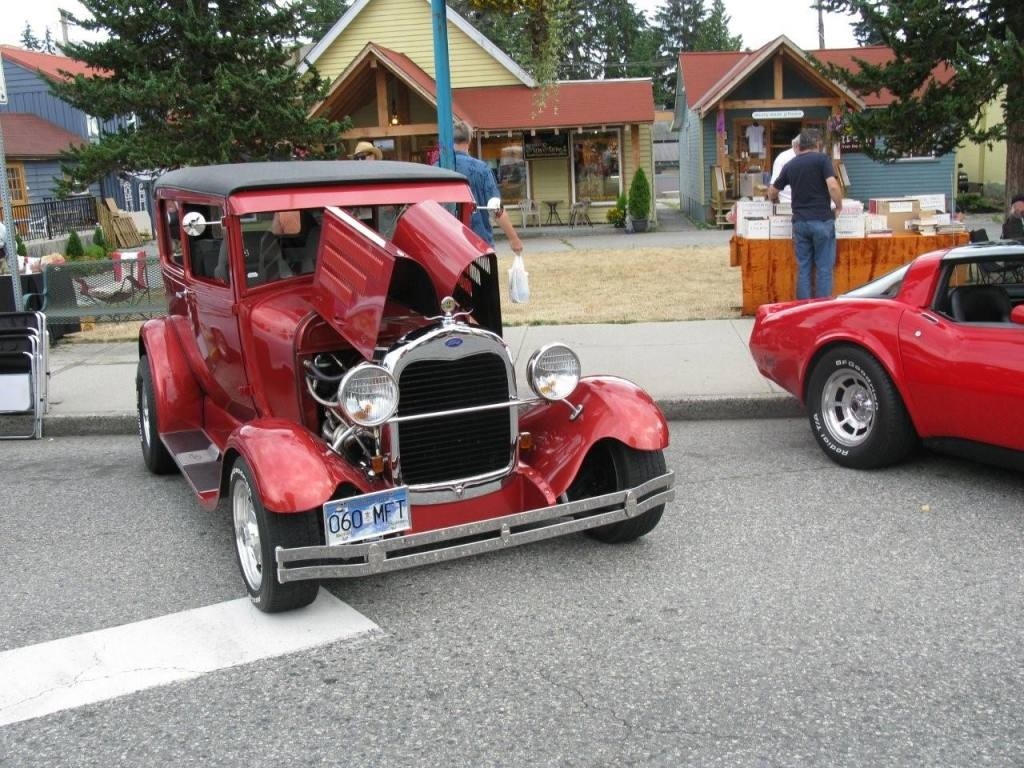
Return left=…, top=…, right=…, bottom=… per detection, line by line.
left=134, top=160, right=677, bottom=615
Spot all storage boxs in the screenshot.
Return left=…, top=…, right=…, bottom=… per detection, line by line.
left=735, top=199, right=951, bottom=240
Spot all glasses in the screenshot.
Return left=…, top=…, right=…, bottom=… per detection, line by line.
left=354, top=153, right=371, bottom=161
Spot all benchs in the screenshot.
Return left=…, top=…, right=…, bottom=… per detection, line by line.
left=23, top=257, right=167, bottom=348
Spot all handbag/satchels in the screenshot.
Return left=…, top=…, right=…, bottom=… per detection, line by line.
left=507, top=254, right=528, bottom=304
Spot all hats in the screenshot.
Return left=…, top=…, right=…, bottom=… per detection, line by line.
left=347, top=142, right=383, bottom=161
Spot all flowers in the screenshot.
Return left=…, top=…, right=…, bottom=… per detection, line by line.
left=825, top=108, right=853, bottom=143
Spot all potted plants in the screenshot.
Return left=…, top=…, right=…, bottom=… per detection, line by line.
left=629, top=168, right=653, bottom=233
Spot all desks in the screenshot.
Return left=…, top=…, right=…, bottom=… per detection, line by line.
left=542, top=200, right=564, bottom=226
left=731, top=233, right=968, bottom=318
left=50, top=211, right=79, bottom=235
left=14, top=219, right=32, bottom=241
left=0, top=272, right=78, bottom=344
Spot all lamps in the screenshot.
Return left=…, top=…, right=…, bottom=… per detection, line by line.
left=391, top=71, right=400, bottom=124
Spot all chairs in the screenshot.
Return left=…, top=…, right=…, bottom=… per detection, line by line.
left=950, top=284, right=1011, bottom=324
left=29, top=216, right=48, bottom=240
left=568, top=197, right=593, bottom=229
left=75, top=250, right=150, bottom=321
left=193, top=227, right=319, bottom=288
left=519, top=199, right=541, bottom=229
left=0, top=310, right=49, bottom=439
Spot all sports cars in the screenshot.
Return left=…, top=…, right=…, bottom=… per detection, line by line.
left=749, top=236, right=1024, bottom=472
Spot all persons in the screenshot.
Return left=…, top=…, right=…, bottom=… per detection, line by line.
left=271, top=211, right=302, bottom=235
left=767, top=127, right=842, bottom=299
left=432, top=121, right=526, bottom=258
left=1000, top=191, right=1023, bottom=240
left=346, top=141, right=394, bottom=219
